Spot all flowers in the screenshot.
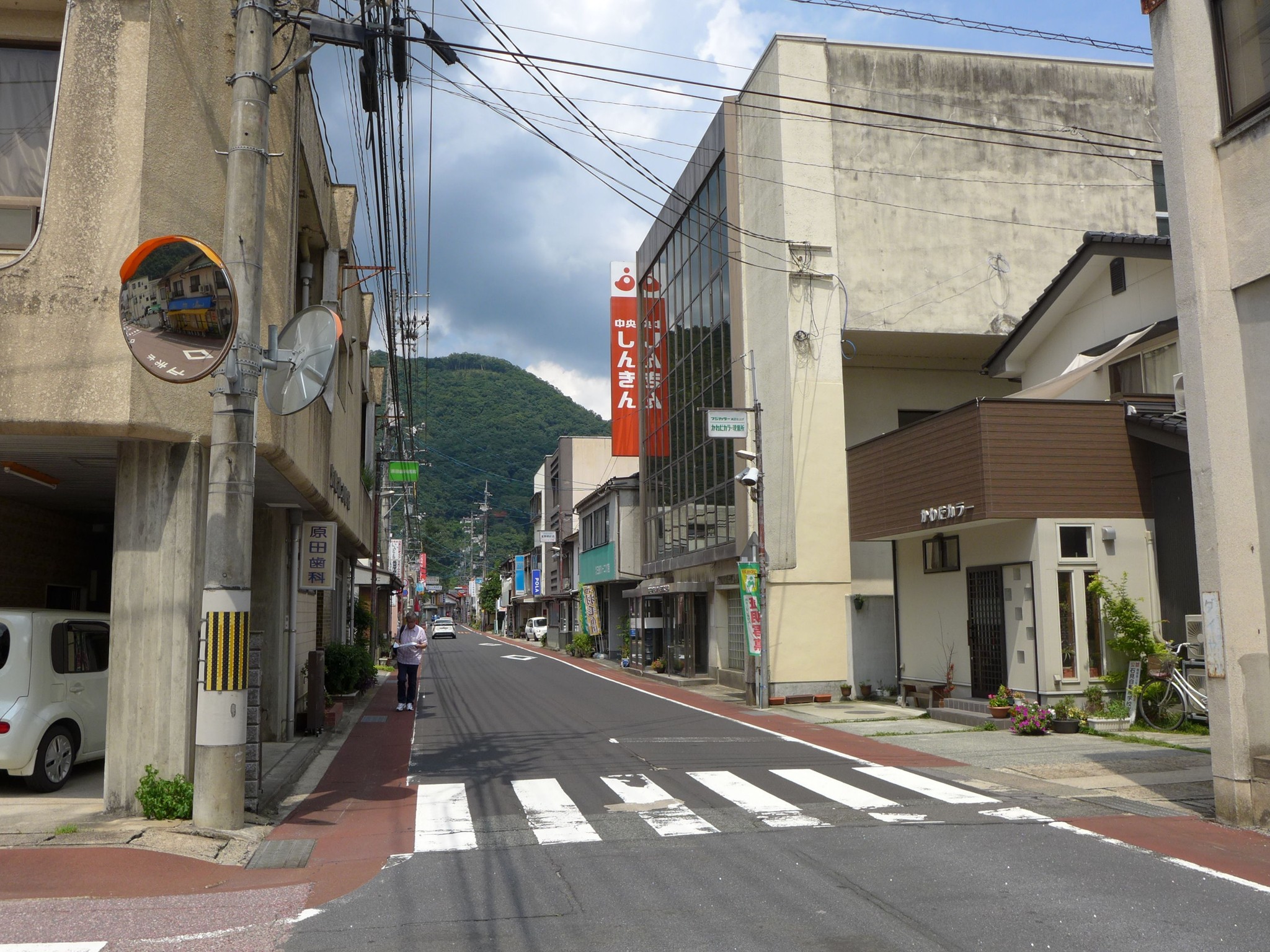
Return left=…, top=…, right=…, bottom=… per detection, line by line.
left=988, top=683, right=1056, bottom=736
left=651, top=655, right=668, bottom=668
left=925, top=609, right=961, bottom=699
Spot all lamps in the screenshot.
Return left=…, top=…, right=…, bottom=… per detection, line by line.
left=0, top=461, right=60, bottom=489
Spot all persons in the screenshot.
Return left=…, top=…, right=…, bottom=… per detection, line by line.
left=394, top=611, right=428, bottom=711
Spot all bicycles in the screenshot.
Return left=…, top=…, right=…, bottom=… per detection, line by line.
left=1138, top=642, right=1208, bottom=731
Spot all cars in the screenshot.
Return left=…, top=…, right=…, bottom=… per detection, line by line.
left=525, top=617, right=547, bottom=641
left=1, top=607, right=110, bottom=794
left=430, top=615, right=457, bottom=639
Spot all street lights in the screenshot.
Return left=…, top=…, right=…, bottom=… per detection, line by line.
left=507, top=553, right=516, bottom=631
left=537, top=519, right=563, bottom=592
left=696, top=403, right=769, bottom=709
left=369, top=452, right=395, bottom=665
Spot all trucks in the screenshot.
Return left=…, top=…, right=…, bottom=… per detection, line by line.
left=147, top=306, right=159, bottom=315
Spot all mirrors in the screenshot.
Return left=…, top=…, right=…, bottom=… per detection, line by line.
left=119, top=242, right=232, bottom=379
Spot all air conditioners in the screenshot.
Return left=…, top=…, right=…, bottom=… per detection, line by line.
left=198, top=285, right=205, bottom=293
left=1173, top=373, right=1185, bottom=412
left=204, top=284, right=213, bottom=293
left=1186, top=668, right=1208, bottom=714
left=1184, top=613, right=1204, bottom=660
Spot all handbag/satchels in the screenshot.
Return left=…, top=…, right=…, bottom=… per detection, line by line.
left=389, top=625, right=406, bottom=656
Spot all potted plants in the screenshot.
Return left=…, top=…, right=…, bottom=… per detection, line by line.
left=617, top=616, right=630, bottom=668
left=852, top=594, right=869, bottom=610
left=874, top=677, right=895, bottom=698
left=378, top=642, right=393, bottom=666
left=565, top=632, right=596, bottom=659
left=1050, top=695, right=1082, bottom=733
left=839, top=681, right=853, bottom=697
left=1089, top=652, right=1099, bottom=677
left=1061, top=639, right=1073, bottom=678
left=1086, top=698, right=1133, bottom=729
left=857, top=677, right=872, bottom=695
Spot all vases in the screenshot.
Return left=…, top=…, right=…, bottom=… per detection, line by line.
left=1006, top=697, right=1014, bottom=706
left=1013, top=697, right=1025, bottom=706
left=987, top=705, right=1011, bottom=719
left=769, top=694, right=833, bottom=706
left=656, top=668, right=665, bottom=674
left=939, top=700, right=944, bottom=707
left=1032, top=720, right=1049, bottom=734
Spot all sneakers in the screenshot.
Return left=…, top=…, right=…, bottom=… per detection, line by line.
left=406, top=702, right=414, bottom=711
left=396, top=703, right=406, bottom=711
